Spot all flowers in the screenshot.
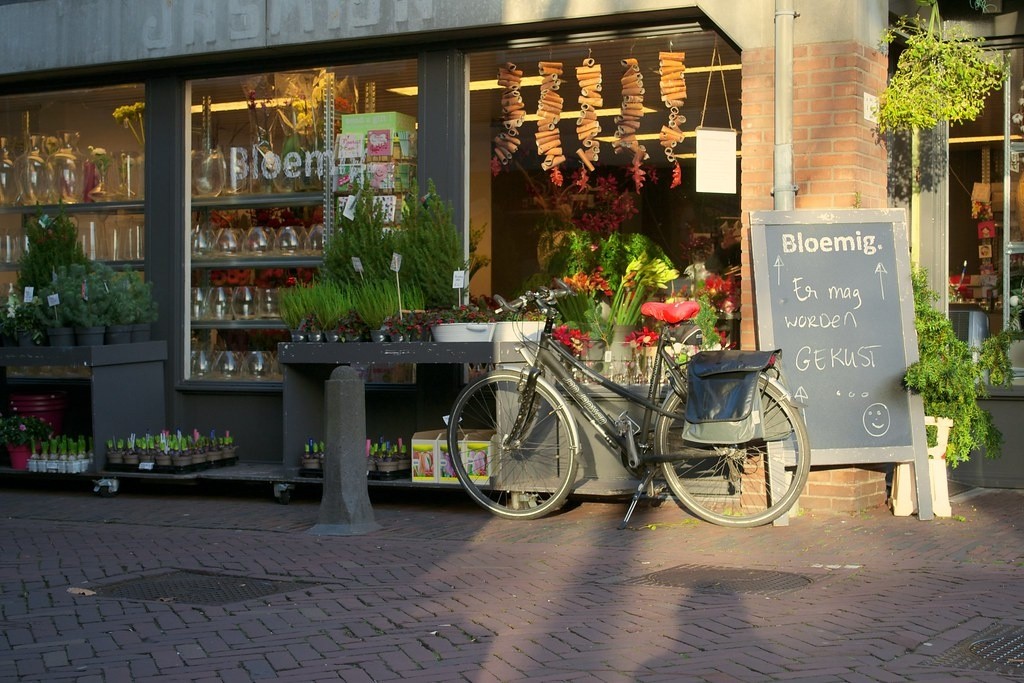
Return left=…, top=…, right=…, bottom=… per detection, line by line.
left=494, top=146, right=740, bottom=362
left=119, top=155, right=134, bottom=186
left=242, top=77, right=362, bottom=179
left=336, top=309, right=369, bottom=337
left=87, top=145, right=113, bottom=176
left=0, top=400, right=56, bottom=451
left=111, top=101, right=144, bottom=152
left=383, top=306, right=548, bottom=336
left=300, top=313, right=321, bottom=334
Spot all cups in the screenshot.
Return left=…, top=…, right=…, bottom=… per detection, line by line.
left=2, top=218, right=143, bottom=263
left=189, top=147, right=323, bottom=378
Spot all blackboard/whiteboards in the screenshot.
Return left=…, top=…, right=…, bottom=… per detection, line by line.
left=750, top=208, right=929, bottom=466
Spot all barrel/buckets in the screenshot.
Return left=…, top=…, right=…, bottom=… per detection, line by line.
left=3, top=391, right=66, bottom=470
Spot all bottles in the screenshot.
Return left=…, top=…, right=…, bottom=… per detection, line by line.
left=0, top=130, right=81, bottom=204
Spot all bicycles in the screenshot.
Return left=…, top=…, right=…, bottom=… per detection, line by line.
left=447, top=280, right=812, bottom=531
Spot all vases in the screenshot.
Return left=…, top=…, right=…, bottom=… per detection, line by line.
left=572, top=322, right=720, bottom=384
left=8, top=395, right=67, bottom=434
left=190, top=140, right=323, bottom=379
left=429, top=323, right=495, bottom=341
left=0, top=129, right=142, bottom=260
left=491, top=322, right=546, bottom=342
left=5, top=440, right=32, bottom=469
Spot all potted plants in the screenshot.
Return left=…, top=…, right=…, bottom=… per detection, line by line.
left=28, top=435, right=96, bottom=475
left=107, top=425, right=240, bottom=474
left=300, top=438, right=325, bottom=475
left=886, top=267, right=1024, bottom=517
left=365, top=433, right=409, bottom=480
left=1, top=209, right=158, bottom=347
left=276, top=173, right=482, bottom=340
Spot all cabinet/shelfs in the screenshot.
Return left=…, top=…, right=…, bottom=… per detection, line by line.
left=558, top=383, right=741, bottom=500
left=0, top=338, right=170, bottom=495
left=983, top=145, right=1024, bottom=310
left=278, top=343, right=562, bottom=505
left=13, top=192, right=322, bottom=331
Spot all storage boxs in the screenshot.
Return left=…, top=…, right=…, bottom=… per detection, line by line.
left=410, top=428, right=496, bottom=481
left=339, top=112, right=420, bottom=189
